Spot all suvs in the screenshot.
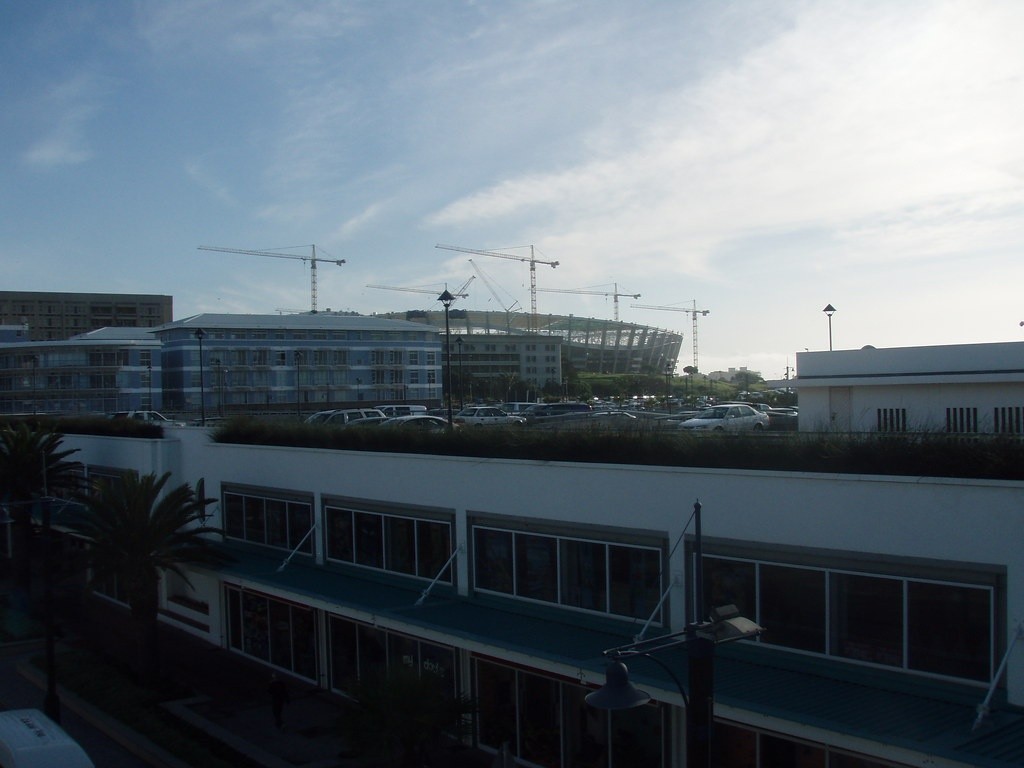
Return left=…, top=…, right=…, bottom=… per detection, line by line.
left=113, top=410, right=186, bottom=427
left=454, top=406, right=528, bottom=426
left=521, top=402, right=593, bottom=420
left=305, top=405, right=461, bottom=438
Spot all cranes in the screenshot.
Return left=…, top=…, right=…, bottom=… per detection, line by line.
left=629, top=299, right=710, bottom=369
left=466, top=258, right=525, bottom=334
left=433, top=241, right=561, bottom=335
left=363, top=276, right=477, bottom=327
left=527, top=281, right=644, bottom=319
left=197, top=243, right=345, bottom=313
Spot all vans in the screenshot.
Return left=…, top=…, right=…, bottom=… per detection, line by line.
left=497, top=403, right=538, bottom=417
left=0, top=709, right=96, bottom=768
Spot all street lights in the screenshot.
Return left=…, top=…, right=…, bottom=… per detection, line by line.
left=296, top=351, right=304, bottom=417
left=147, top=364, right=153, bottom=409
left=456, top=336, right=465, bottom=411
left=822, top=303, right=837, bottom=350
left=216, top=358, right=224, bottom=416
left=435, top=289, right=458, bottom=433
left=666, top=363, right=673, bottom=414
left=194, top=328, right=209, bottom=426
left=32, top=355, right=38, bottom=418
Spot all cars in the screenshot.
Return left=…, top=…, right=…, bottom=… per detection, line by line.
left=676, top=404, right=771, bottom=433
left=717, top=397, right=798, bottom=427
left=591, top=411, right=636, bottom=420
